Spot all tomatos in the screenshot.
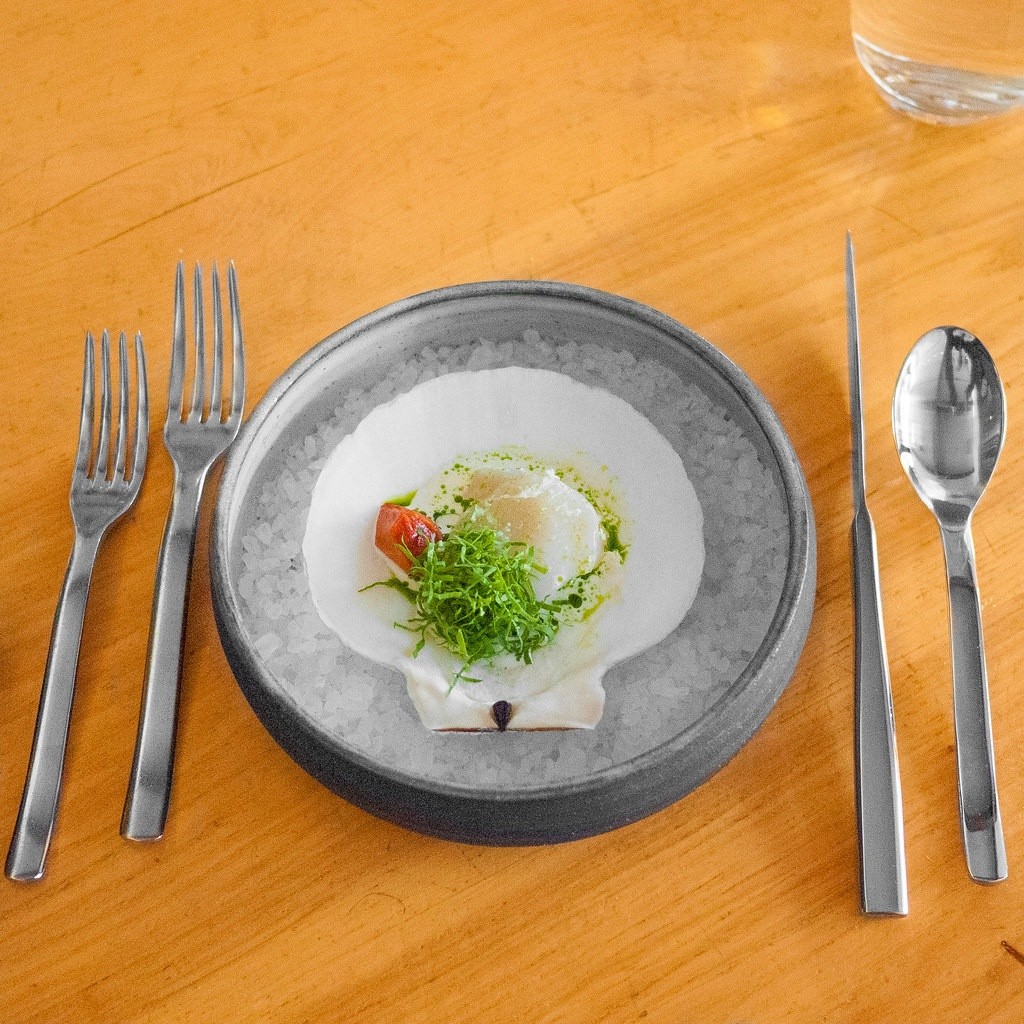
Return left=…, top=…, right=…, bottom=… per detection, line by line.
left=376, top=504, right=443, bottom=572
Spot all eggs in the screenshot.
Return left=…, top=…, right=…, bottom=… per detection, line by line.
left=411, top=448, right=602, bottom=598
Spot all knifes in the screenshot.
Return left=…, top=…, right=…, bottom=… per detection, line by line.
left=843, top=224, right=910, bottom=917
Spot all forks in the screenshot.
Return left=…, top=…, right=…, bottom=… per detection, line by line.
left=5, top=328, right=151, bottom=887
left=117, top=254, right=246, bottom=846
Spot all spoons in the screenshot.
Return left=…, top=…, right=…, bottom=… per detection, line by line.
left=894, top=326, right=1006, bottom=884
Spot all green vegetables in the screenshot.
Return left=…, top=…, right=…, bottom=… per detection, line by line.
left=357, top=528, right=560, bottom=692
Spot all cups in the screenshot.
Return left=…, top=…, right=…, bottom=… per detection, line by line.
left=850, top=0, right=1024, bottom=127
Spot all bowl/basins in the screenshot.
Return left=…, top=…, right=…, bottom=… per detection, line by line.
left=210, top=279, right=816, bottom=845
